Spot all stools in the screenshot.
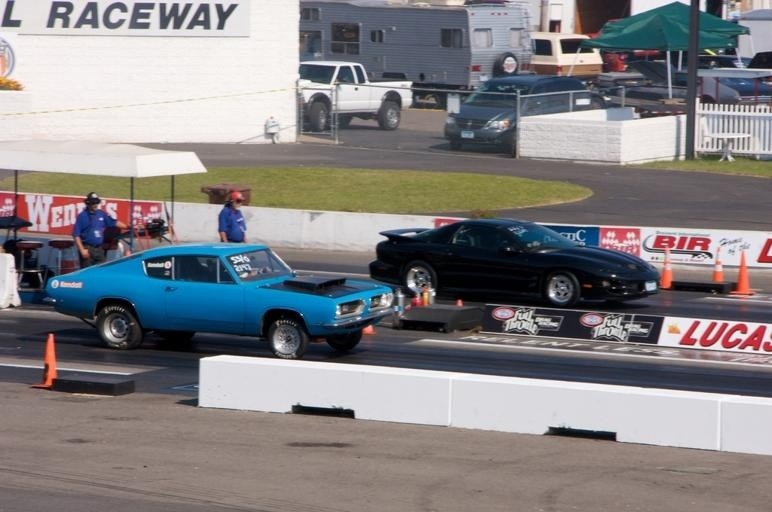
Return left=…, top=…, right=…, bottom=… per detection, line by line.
left=17, top=241, right=43, bottom=287
left=49, top=240, right=76, bottom=273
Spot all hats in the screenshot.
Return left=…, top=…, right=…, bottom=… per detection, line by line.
left=88, top=192, right=98, bottom=199
left=230, top=192, right=246, bottom=201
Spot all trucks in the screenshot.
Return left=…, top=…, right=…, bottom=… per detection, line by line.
left=299, top=0, right=534, bottom=110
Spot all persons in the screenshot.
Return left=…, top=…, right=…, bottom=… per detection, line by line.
left=219, top=192, right=248, bottom=243
left=72, top=192, right=133, bottom=269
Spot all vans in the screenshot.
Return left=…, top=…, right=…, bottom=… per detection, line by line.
left=445, top=75, right=591, bottom=152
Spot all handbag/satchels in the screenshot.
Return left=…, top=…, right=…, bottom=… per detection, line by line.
left=102, top=227, right=121, bottom=250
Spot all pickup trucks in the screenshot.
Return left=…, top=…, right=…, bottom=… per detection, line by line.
left=299, top=61, right=414, bottom=129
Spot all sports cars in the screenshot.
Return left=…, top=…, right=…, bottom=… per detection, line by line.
left=42, top=240, right=393, bottom=360
left=367, top=220, right=661, bottom=309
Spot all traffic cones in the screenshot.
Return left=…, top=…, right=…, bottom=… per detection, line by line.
left=729, top=251, right=753, bottom=296
left=32, top=334, right=60, bottom=389
left=659, top=248, right=673, bottom=290
left=711, top=248, right=726, bottom=283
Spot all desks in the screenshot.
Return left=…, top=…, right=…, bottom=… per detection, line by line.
left=201, top=183, right=251, bottom=205
left=710, top=134, right=750, bottom=162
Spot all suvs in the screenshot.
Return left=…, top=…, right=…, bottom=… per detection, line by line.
left=526, top=19, right=771, bottom=116
